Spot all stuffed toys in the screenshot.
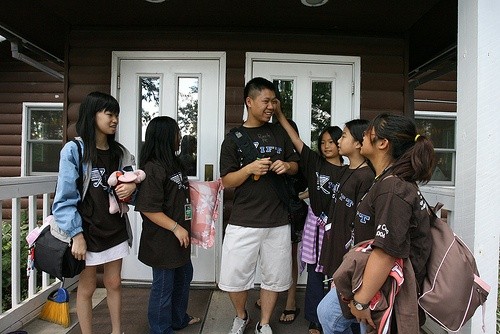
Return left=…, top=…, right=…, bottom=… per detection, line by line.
left=108, top=168, right=146, bottom=218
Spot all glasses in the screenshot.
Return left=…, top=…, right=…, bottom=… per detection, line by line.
left=362, top=130, right=375, bottom=137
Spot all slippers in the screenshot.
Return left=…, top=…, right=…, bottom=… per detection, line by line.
left=279, top=307, right=300, bottom=323
left=186, top=314, right=200, bottom=326
left=256, top=299, right=262, bottom=309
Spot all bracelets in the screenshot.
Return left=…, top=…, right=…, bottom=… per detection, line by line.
left=171, top=222, right=177, bottom=231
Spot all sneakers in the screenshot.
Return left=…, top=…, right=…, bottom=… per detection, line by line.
left=255, top=322, right=272, bottom=334
left=230, top=311, right=248, bottom=334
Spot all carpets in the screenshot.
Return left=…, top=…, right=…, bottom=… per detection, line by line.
left=66, top=287, right=214, bottom=334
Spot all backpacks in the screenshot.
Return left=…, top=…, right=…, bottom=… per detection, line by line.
left=417, top=186, right=490, bottom=333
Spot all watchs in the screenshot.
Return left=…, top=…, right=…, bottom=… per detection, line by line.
left=352, top=296, right=370, bottom=311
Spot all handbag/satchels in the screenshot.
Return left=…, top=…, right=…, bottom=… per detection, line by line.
left=34, top=226, right=85, bottom=279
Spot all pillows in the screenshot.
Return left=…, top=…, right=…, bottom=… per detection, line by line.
left=187, top=178, right=224, bottom=250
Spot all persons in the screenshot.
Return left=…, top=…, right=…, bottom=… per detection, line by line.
left=178, top=135, right=197, bottom=176
left=134, top=116, right=202, bottom=334
left=219, top=77, right=301, bottom=334
left=255, top=109, right=434, bottom=334
left=53, top=90, right=139, bottom=334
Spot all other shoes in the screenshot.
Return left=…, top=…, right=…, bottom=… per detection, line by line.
left=308, top=321, right=321, bottom=334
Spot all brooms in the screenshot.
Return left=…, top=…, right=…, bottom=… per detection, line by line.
left=40, top=277, right=70, bottom=328
